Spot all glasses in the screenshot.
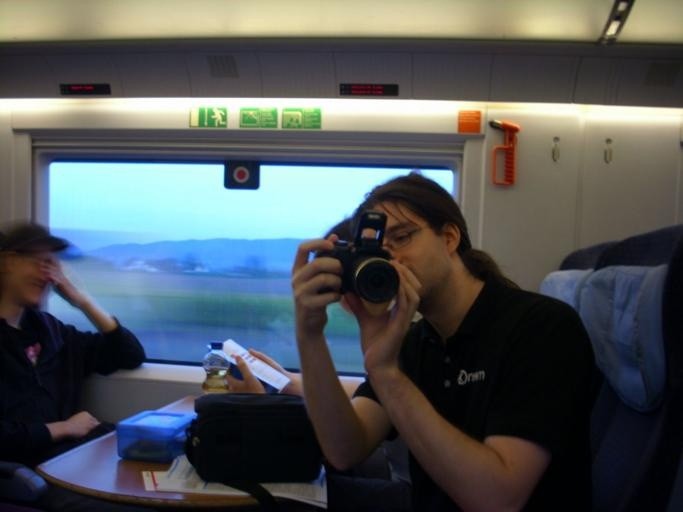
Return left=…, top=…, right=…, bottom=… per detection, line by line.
left=382, top=223, right=429, bottom=252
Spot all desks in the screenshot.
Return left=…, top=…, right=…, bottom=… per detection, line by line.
left=32, top=391, right=263, bottom=509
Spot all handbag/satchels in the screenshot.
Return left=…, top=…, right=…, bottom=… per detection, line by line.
left=184, top=392, right=324, bottom=485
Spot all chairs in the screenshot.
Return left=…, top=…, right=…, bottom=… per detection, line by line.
left=538, top=224, right=682, bottom=505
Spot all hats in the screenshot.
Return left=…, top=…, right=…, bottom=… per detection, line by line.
left=2, top=224, right=68, bottom=256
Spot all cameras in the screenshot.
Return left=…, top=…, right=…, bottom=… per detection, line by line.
left=314, top=209, right=400, bottom=305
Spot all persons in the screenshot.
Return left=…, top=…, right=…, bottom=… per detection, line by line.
left=223, top=215, right=424, bottom=511
left=1, top=221, right=166, bottom=512
left=290, top=172, right=598, bottom=511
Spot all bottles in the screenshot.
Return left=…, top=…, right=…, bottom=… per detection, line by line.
left=199, top=342, right=231, bottom=395
